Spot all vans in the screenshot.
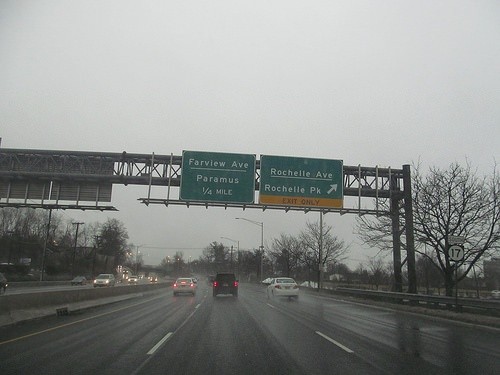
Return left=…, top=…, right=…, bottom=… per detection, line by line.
left=211, top=272, right=239, bottom=298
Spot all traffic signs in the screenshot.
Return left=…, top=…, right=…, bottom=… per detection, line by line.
left=179, top=150, right=256, bottom=204
left=259, top=154, right=344, bottom=209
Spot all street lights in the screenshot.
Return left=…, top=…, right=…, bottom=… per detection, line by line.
left=190, top=256, right=191, bottom=270
left=236, top=217, right=263, bottom=282
left=221, top=236, right=240, bottom=272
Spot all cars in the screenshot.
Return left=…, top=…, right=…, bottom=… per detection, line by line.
left=71, top=275, right=88, bottom=287
left=207, top=274, right=217, bottom=286
left=173, top=277, right=197, bottom=296
left=116, top=274, right=159, bottom=284
left=93, top=273, right=116, bottom=287
left=0, top=272, right=9, bottom=294
left=262, top=278, right=273, bottom=284
left=266, top=277, right=300, bottom=300
